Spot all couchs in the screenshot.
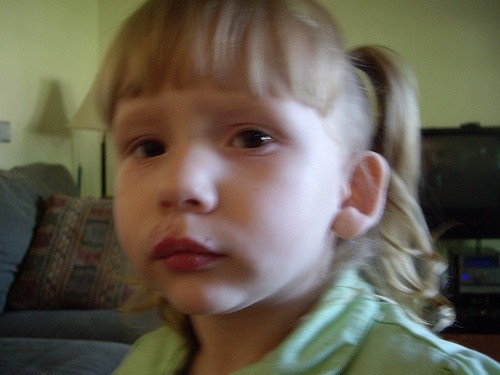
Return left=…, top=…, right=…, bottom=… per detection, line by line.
left=1, top=163, right=167, bottom=375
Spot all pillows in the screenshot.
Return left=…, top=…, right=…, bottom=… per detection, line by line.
left=5, top=194, right=129, bottom=310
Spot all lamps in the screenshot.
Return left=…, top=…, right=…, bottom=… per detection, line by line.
left=64, top=67, right=116, bottom=197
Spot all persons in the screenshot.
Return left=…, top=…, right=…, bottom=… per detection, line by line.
left=98, top=0, right=499, bottom=375
